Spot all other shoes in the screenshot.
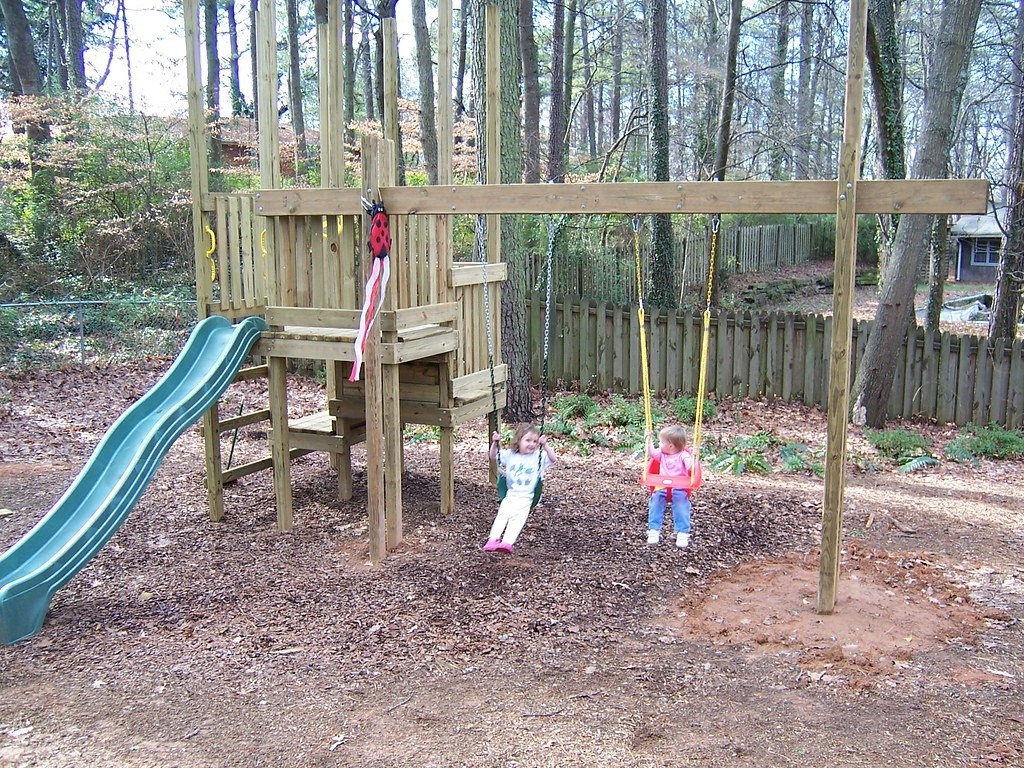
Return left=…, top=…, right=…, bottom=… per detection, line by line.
left=483, top=541, right=498, bottom=552
left=647, top=529, right=660, bottom=544
left=496, top=542, right=513, bottom=554
left=676, top=532, right=692, bottom=548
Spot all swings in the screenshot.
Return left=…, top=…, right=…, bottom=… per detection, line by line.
left=634, top=232, right=716, bottom=501
left=478, top=219, right=554, bottom=509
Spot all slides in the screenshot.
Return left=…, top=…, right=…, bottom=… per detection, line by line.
left=0, top=314, right=269, bottom=646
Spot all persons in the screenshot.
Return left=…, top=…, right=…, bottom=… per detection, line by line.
left=483, top=423, right=557, bottom=553
left=645, top=425, right=701, bottom=547
